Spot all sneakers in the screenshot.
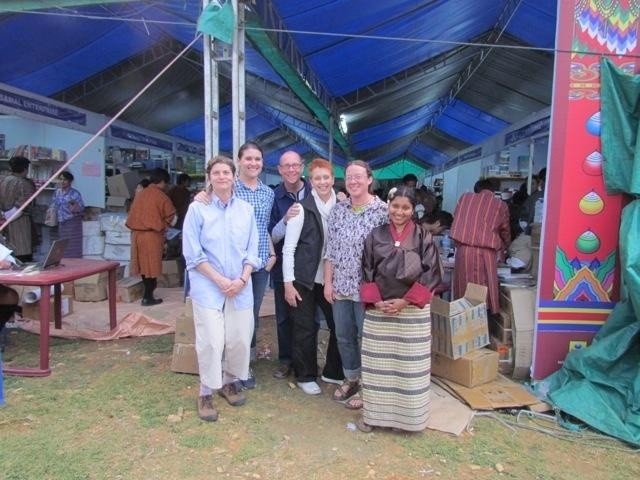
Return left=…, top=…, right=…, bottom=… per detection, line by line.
left=218, top=381, right=247, bottom=406
left=197, top=393, right=218, bottom=420
left=242, top=359, right=345, bottom=395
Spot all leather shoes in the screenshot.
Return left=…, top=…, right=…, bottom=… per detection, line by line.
left=140, top=298, right=162, bottom=306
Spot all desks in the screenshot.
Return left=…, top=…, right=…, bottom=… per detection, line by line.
left=0, top=258, right=120, bottom=378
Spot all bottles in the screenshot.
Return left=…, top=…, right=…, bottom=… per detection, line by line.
left=441, top=234, right=451, bottom=257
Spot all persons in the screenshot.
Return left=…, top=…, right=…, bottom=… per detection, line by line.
left=127, top=142, right=548, bottom=435
left=1, top=243, right=19, bottom=333
left=46, top=171, right=85, bottom=258
left=0, top=157, right=35, bottom=260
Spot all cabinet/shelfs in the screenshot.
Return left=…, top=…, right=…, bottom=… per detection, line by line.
left=1, top=130, right=205, bottom=263
left=409, top=132, right=555, bottom=264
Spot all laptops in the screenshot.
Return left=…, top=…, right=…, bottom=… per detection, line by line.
left=11, top=238, right=69, bottom=270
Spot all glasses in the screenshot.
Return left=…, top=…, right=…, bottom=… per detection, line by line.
left=279, top=163, right=301, bottom=170
left=343, top=175, right=365, bottom=181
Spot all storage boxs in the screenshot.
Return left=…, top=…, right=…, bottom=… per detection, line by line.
left=430, top=266, right=537, bottom=392
left=23, top=264, right=201, bottom=410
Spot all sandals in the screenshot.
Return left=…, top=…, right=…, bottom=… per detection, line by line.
left=335, top=382, right=373, bottom=432
